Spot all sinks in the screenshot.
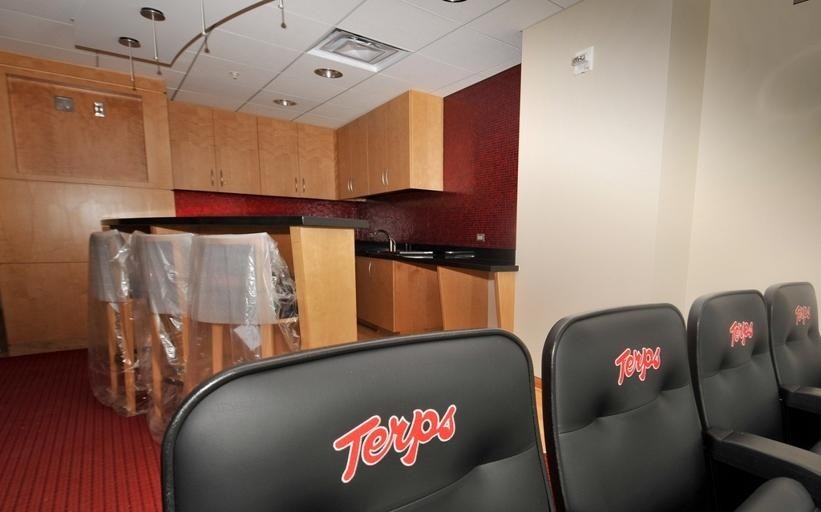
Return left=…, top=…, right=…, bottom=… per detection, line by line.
left=399, top=249, right=434, bottom=254
left=402, top=254, right=434, bottom=259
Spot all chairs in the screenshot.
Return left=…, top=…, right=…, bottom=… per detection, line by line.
left=159, top=281, right=816, bottom=511
left=83, top=226, right=296, bottom=438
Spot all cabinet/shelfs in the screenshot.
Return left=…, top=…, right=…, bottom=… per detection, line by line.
left=355, top=255, right=393, bottom=330
left=333, top=118, right=367, bottom=197
left=167, top=102, right=260, bottom=193
left=365, top=90, right=408, bottom=194
left=261, top=111, right=336, bottom=199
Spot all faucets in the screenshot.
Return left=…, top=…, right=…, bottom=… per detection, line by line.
left=374, top=228, right=397, bottom=252
